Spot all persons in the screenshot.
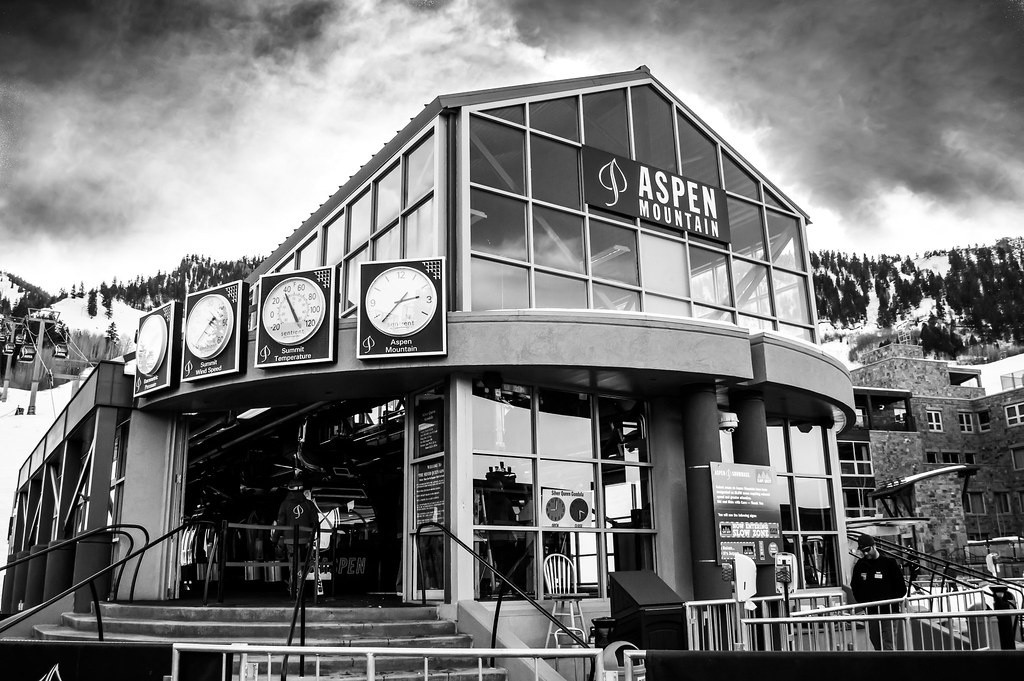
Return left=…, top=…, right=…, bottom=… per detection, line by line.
left=850, top=534, right=906, bottom=650
left=902, top=548, right=922, bottom=594
left=943, top=565, right=958, bottom=592
left=270, top=479, right=319, bottom=606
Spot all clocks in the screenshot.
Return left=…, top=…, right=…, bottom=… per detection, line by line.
left=542, top=488, right=594, bottom=528
left=365, top=267, right=438, bottom=335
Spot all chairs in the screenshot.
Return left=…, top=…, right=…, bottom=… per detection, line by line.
left=554, top=627, right=586, bottom=681
left=542, top=554, right=590, bottom=648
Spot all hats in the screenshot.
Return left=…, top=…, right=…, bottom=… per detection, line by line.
left=858, top=534, right=874, bottom=550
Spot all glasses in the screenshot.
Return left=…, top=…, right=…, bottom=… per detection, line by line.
left=860, top=546, right=871, bottom=553
